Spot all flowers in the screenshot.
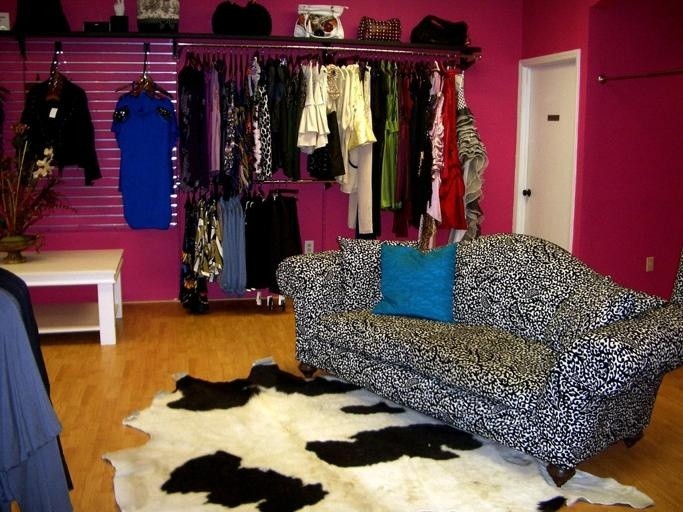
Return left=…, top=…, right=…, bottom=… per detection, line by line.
left=0, top=123, right=78, bottom=252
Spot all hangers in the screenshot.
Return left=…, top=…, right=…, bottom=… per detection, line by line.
left=179, top=41, right=486, bottom=85
left=36, top=66, right=70, bottom=85
left=115, top=66, right=172, bottom=100
left=179, top=177, right=297, bottom=211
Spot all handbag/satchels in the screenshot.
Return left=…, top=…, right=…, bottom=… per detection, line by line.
left=354, top=13, right=403, bottom=45
left=82, top=20, right=111, bottom=36
left=134, top=0, right=185, bottom=36
left=292, top=3, right=349, bottom=41
left=207, top=0, right=275, bottom=40
left=407, top=12, right=471, bottom=49
left=9, top=0, right=71, bottom=39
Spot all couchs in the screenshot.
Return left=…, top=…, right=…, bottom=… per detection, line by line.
left=271, top=232, right=683, bottom=485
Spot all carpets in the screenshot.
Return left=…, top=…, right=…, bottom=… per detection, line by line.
left=97, top=354, right=658, bottom=510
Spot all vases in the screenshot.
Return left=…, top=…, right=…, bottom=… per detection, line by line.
left=0, top=235, right=37, bottom=264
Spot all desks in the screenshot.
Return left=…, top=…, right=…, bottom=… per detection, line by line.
left=0, top=248, right=126, bottom=348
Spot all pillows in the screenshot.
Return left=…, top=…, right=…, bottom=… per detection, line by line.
left=542, top=268, right=669, bottom=355
left=375, top=239, right=459, bottom=322
left=334, top=230, right=418, bottom=313
